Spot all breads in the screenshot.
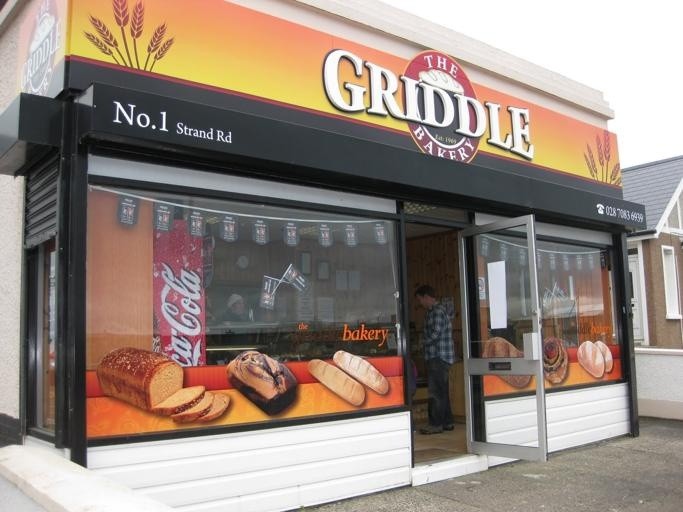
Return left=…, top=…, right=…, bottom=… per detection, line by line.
left=543, top=336, right=568, bottom=383
left=482, top=337, right=532, bottom=388
left=307, top=357, right=366, bottom=406
left=333, top=350, right=390, bottom=394
left=577, top=340, right=605, bottom=377
left=226, top=349, right=298, bottom=416
left=97, top=347, right=231, bottom=423
left=593, top=341, right=614, bottom=373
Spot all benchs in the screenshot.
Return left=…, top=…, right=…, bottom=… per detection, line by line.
left=206, top=345, right=267, bottom=364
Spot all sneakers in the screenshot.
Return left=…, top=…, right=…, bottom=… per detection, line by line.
left=420, top=423, right=454, bottom=434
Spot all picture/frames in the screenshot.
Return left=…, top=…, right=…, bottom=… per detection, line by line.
left=301, top=251, right=312, bottom=275
left=317, top=261, right=331, bottom=281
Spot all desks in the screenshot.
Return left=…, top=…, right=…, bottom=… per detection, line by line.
left=207, top=321, right=298, bottom=344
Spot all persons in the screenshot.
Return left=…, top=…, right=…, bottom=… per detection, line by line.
left=213, top=294, right=256, bottom=356
left=414, top=285, right=455, bottom=435
